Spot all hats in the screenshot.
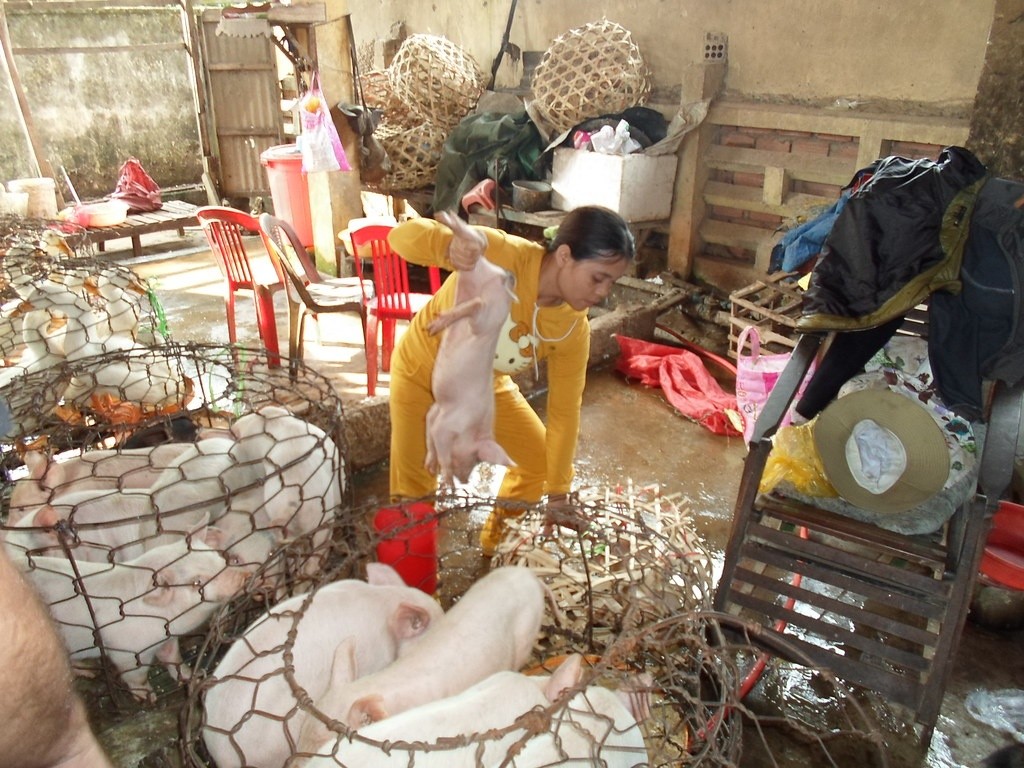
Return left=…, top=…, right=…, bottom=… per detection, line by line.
left=813, top=389, right=951, bottom=513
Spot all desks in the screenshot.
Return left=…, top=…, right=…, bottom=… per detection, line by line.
left=465, top=204, right=662, bottom=279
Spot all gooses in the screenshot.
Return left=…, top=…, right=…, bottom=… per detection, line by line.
left=0, top=226, right=179, bottom=463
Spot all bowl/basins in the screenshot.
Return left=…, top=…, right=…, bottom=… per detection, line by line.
left=50, top=207, right=89, bottom=232
left=82, top=201, right=130, bottom=227
left=338, top=229, right=394, bottom=257
left=980, top=500, right=1024, bottom=589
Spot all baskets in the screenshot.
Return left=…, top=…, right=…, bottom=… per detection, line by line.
left=357, top=19, right=654, bottom=192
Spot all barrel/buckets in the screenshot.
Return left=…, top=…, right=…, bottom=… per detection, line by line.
left=0, top=191, right=29, bottom=217
left=511, top=180, right=552, bottom=213
left=375, top=502, right=439, bottom=594
left=260, top=144, right=313, bottom=247
left=9, top=177, right=59, bottom=220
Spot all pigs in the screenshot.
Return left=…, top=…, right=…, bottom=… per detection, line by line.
left=308, top=652, right=650, bottom=768
left=419, top=208, right=519, bottom=493
left=199, top=562, right=446, bottom=768
left=0, top=402, right=346, bottom=703
left=282, top=564, right=564, bottom=767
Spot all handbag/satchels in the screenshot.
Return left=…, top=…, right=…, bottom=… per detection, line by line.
left=298, top=70, right=353, bottom=174
left=359, top=134, right=391, bottom=182
left=110, top=156, right=163, bottom=212
left=338, top=101, right=384, bottom=135
left=736, top=327, right=817, bottom=450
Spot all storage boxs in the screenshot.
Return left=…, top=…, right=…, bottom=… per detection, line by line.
left=552, top=148, right=678, bottom=225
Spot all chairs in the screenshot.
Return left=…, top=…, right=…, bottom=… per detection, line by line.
left=197, top=206, right=435, bottom=397
left=711, top=158, right=1008, bottom=751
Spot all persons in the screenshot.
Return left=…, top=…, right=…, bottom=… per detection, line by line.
left=387, top=205, right=637, bottom=607
